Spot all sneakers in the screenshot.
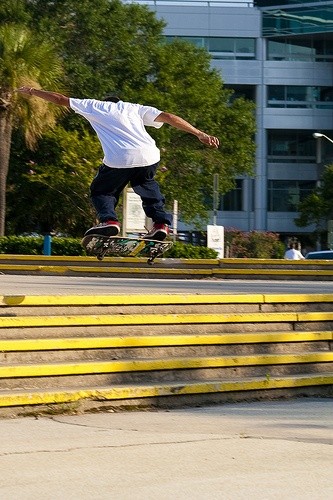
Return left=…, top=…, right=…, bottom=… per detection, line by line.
left=84, top=220, right=121, bottom=236
left=141, top=222, right=169, bottom=241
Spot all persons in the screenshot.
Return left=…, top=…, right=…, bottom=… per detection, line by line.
left=283, top=243, right=304, bottom=259
left=19, top=86, right=219, bottom=242
left=289, top=237, right=300, bottom=252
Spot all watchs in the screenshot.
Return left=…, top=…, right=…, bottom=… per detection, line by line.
left=28, top=88, right=34, bottom=96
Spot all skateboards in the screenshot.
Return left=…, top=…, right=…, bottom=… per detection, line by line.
left=80, top=233, right=174, bottom=266
